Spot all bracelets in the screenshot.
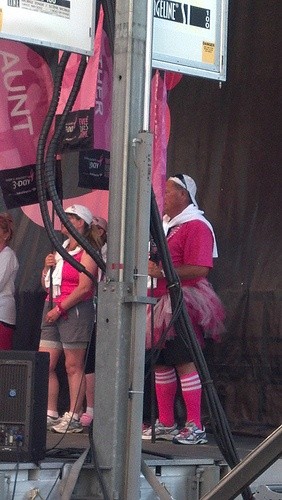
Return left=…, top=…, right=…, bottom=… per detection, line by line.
left=56, top=303, right=65, bottom=316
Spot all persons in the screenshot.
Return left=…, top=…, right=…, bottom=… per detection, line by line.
left=38, top=205, right=97, bottom=433
left=0, top=212, right=19, bottom=350
left=142, top=174, right=224, bottom=445
left=80, top=217, right=107, bottom=426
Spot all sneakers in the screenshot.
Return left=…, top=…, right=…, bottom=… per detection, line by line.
left=51, top=412, right=84, bottom=433
left=172, top=421, right=208, bottom=445
left=142, top=418, right=180, bottom=441
left=46, top=414, right=62, bottom=431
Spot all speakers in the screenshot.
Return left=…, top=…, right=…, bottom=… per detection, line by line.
left=1, top=349, right=50, bottom=464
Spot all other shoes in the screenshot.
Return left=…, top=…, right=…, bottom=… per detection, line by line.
left=80, top=412, right=92, bottom=426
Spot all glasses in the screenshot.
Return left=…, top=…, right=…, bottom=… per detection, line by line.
left=171, top=172, right=187, bottom=189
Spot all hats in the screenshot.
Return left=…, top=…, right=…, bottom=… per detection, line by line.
left=65, top=204, right=93, bottom=229
left=168, top=174, right=199, bottom=207
left=91, top=216, right=107, bottom=232
